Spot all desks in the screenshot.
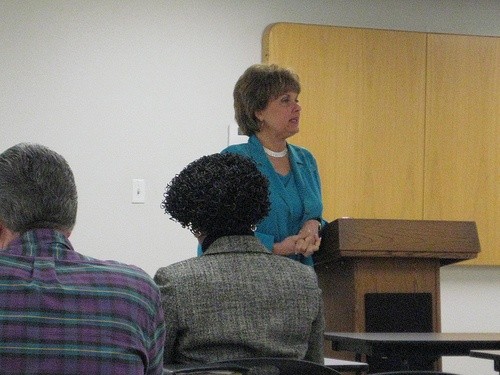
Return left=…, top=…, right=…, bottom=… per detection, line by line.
left=309, top=216, right=483, bottom=374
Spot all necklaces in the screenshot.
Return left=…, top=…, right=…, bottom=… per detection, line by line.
left=262, top=146, right=287, bottom=157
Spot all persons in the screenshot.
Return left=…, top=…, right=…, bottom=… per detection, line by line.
left=195, top=62, right=327, bottom=272
left=0, top=140, right=165, bottom=375
left=151, top=151, right=324, bottom=375
